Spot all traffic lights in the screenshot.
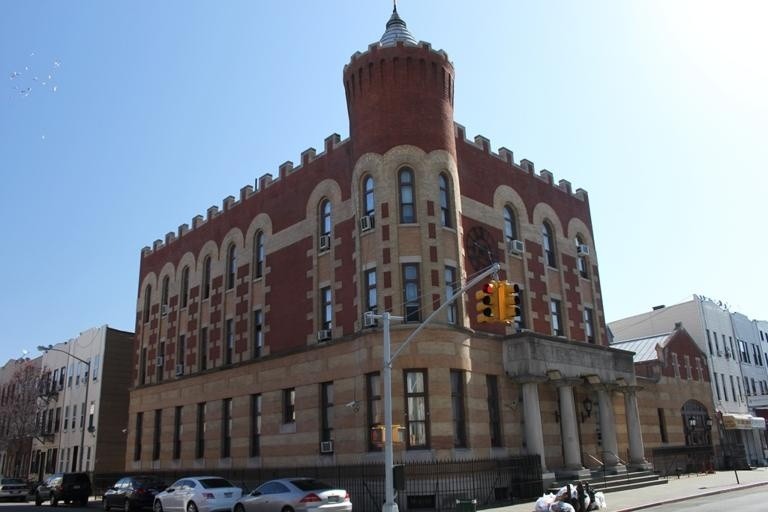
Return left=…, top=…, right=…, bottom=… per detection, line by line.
left=482, top=279, right=498, bottom=323
left=499, top=282, right=521, bottom=324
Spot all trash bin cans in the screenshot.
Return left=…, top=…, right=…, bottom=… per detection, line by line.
left=456, top=498, right=478, bottom=511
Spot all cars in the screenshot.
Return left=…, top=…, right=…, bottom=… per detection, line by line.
left=230, top=476, right=353, bottom=512
left=0, top=477, right=30, bottom=502
left=102, top=475, right=167, bottom=512
left=34, top=471, right=91, bottom=506
left=152, top=476, right=244, bottom=512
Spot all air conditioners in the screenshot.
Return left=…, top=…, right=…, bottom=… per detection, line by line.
left=577, top=243, right=591, bottom=257
left=318, top=214, right=372, bottom=252
left=317, top=310, right=377, bottom=342
left=155, top=304, right=183, bottom=377
left=319, top=440, right=334, bottom=455
left=509, top=239, right=526, bottom=256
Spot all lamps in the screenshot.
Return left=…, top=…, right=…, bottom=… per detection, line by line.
left=687, top=415, right=713, bottom=435
left=581, top=394, right=592, bottom=420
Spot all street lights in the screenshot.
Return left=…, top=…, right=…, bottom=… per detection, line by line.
left=35, top=345, right=91, bottom=471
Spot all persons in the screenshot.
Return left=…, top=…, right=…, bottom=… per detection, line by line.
left=555, top=481, right=595, bottom=512
